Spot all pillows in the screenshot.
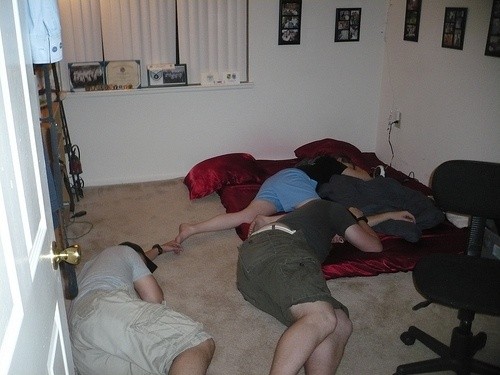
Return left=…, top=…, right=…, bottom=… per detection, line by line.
left=183, top=150, right=267, bottom=200
left=293, top=136, right=362, bottom=165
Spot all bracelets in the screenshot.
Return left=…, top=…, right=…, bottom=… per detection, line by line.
left=152, top=244, right=162, bottom=255
left=353, top=164, right=357, bottom=169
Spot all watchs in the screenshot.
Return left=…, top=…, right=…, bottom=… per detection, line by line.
left=357, top=216, right=368, bottom=222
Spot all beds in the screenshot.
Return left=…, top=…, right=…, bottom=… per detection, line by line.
left=204, top=151, right=436, bottom=281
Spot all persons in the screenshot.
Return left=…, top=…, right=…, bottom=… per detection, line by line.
left=73, top=66, right=102, bottom=82
left=284, top=8, right=298, bottom=15
left=237, top=201, right=416, bottom=375
left=282, top=30, right=295, bottom=41
left=174, top=152, right=369, bottom=256
left=164, top=72, right=182, bottom=83
left=406, top=25, right=415, bottom=36
left=68, top=240, right=216, bottom=375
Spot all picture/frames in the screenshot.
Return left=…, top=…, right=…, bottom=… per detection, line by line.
left=277, top=0, right=303, bottom=45
left=441, top=6, right=467, bottom=50
left=334, top=7, right=362, bottom=42
left=147, top=64, right=189, bottom=87
left=484, top=0, right=500, bottom=58
left=68, top=60, right=104, bottom=92
left=402, top=0, right=423, bottom=43
left=106, top=59, right=141, bottom=89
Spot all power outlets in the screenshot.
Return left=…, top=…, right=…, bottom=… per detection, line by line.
left=391, top=112, right=401, bottom=128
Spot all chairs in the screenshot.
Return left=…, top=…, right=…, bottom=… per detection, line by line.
left=396, top=160, right=500, bottom=375
